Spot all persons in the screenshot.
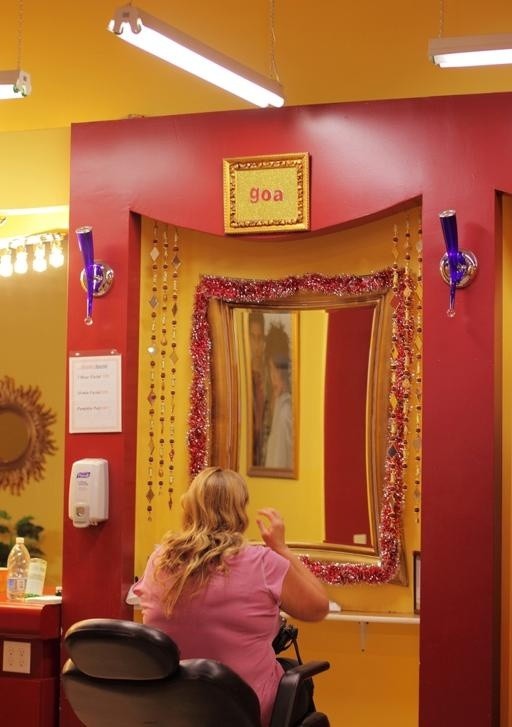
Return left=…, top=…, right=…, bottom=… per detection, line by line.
left=265, top=323, right=294, bottom=466
left=248, top=313, right=265, bottom=465
left=126, top=466, right=329, bottom=727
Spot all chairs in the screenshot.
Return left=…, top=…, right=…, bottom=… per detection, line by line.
left=60, top=618, right=336, bottom=726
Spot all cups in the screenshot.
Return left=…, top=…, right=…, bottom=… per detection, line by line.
left=0, top=567, right=10, bottom=601
left=25, top=555, right=48, bottom=597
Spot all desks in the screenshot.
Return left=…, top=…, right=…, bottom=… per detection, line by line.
left=277, top=610, right=421, bottom=651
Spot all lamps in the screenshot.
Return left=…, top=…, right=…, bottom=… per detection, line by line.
left=432, top=207, right=479, bottom=318
left=0, top=1, right=33, bottom=104
left=425, top=0, right=512, bottom=71
left=72, top=223, right=116, bottom=327
left=103, top=0, right=288, bottom=113
left=3, top=229, right=70, bottom=281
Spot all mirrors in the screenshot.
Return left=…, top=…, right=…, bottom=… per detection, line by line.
left=182, top=267, right=414, bottom=586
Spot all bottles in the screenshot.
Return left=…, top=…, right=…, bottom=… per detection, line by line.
left=56, top=585, right=63, bottom=594
left=7, top=537, right=31, bottom=601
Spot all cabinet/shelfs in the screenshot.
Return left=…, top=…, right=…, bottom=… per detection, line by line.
left=1, top=599, right=64, bottom=724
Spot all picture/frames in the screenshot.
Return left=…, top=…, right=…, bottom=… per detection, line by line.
left=232, top=307, right=302, bottom=483
